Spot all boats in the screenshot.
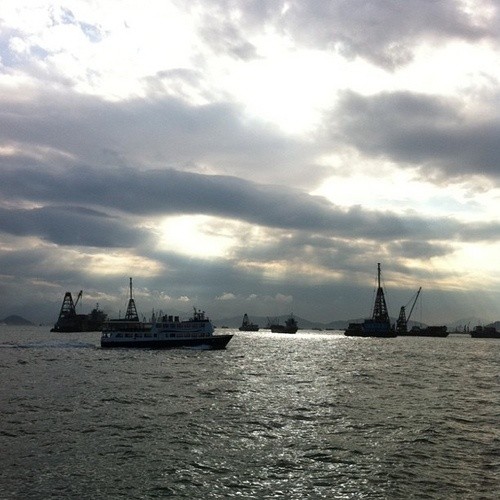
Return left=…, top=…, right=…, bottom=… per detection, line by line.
left=50, top=277, right=139, bottom=333
left=239, top=313, right=259, bottom=331
left=100, top=305, right=234, bottom=350
left=420, top=325, right=449, bottom=337
left=344, top=262, right=421, bottom=337
left=271, top=318, right=299, bottom=334
left=470, top=326, right=500, bottom=338
